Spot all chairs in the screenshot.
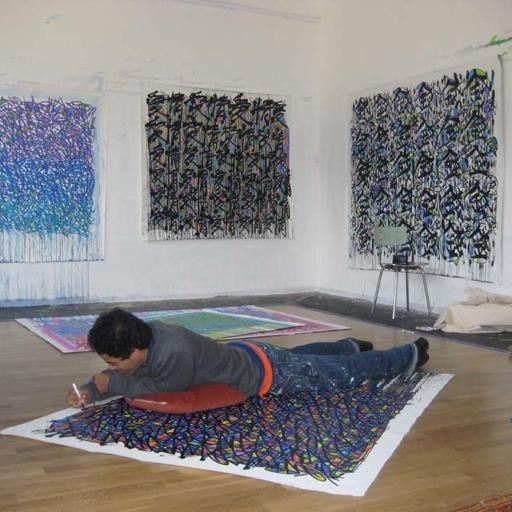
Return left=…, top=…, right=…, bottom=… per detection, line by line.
left=371, top=227, right=432, bottom=320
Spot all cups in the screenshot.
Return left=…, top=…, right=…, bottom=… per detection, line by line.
left=392, top=254, right=408, bottom=265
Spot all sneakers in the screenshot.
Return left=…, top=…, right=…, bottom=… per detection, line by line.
left=347, top=338, right=372, bottom=352
left=414, top=337, right=429, bottom=366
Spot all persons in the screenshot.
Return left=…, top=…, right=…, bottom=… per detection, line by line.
left=67, top=307, right=429, bottom=408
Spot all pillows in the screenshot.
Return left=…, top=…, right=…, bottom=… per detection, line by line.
left=124, top=383, right=247, bottom=414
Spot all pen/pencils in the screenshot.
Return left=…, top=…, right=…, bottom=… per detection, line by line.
left=72, top=383, right=86, bottom=410
